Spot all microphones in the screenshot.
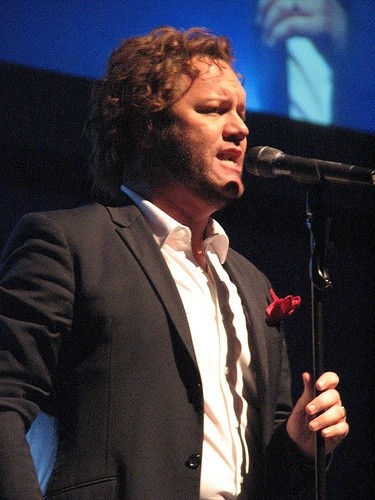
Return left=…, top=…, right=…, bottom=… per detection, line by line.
left=244, top=146, right=375, bottom=186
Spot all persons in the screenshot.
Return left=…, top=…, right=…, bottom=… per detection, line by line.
left=254, top=1, right=375, bottom=132
left=1, top=26, right=349, bottom=500
